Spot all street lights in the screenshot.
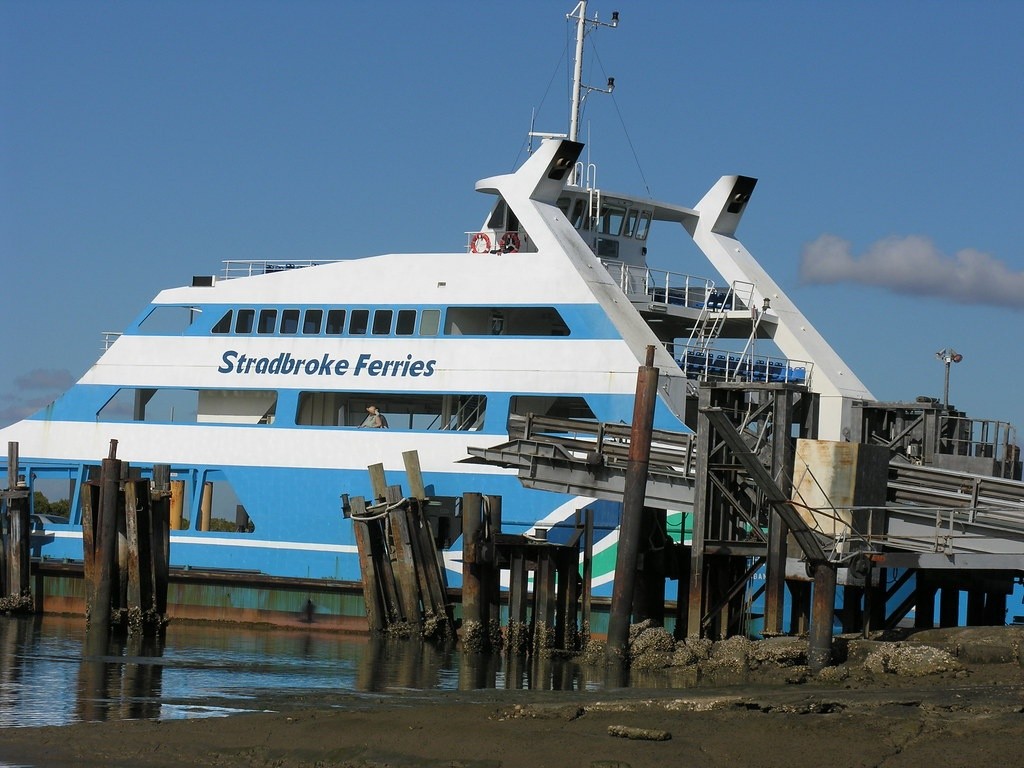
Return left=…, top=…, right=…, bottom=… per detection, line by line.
left=935, top=345, right=964, bottom=411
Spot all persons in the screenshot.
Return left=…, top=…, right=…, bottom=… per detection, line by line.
left=490, top=308, right=505, bottom=335
left=364, top=406, right=389, bottom=429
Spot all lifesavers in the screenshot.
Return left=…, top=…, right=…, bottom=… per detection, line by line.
left=471, top=232, right=491, bottom=254
left=499, top=231, right=520, bottom=253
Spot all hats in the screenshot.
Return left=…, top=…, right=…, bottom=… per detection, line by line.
left=366, top=406, right=376, bottom=414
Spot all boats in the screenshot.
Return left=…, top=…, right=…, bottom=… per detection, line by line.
left=0, top=0, right=1023, bottom=654
